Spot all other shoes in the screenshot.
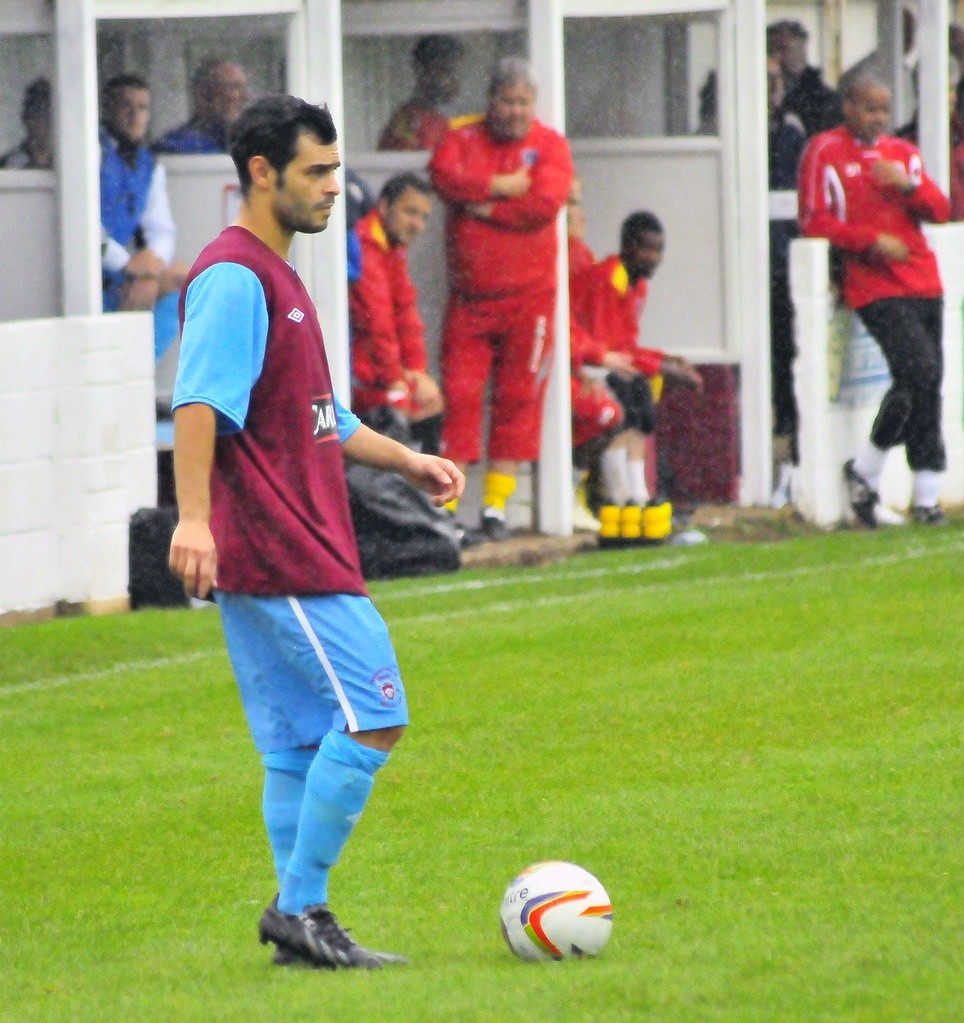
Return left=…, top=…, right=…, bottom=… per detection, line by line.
left=483, top=511, right=505, bottom=541
left=841, top=457, right=878, bottom=526
left=911, top=506, right=947, bottom=522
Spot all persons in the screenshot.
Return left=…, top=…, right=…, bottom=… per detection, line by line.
left=377, top=35, right=463, bottom=152
left=428, top=58, right=573, bottom=548
left=101, top=73, right=189, bottom=313
left=768, top=5, right=964, bottom=467
left=567, top=181, right=704, bottom=511
left=147, top=59, right=252, bottom=154
left=348, top=171, right=445, bottom=455
left=167, top=98, right=467, bottom=969
left=797, top=67, right=954, bottom=528
left=1, top=77, right=56, bottom=167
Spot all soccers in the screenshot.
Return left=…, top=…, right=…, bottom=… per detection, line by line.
left=498, top=858, right=615, bottom=964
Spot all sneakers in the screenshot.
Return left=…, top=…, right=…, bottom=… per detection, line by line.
left=257, top=891, right=402, bottom=970
